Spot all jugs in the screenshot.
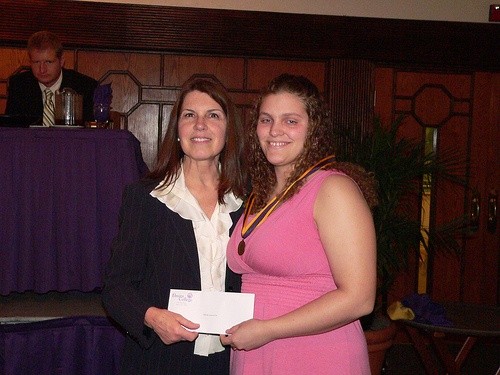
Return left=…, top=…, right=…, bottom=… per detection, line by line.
left=55, top=88, right=81, bottom=126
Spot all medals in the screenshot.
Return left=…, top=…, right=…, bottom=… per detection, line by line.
left=238, top=240, right=246, bottom=255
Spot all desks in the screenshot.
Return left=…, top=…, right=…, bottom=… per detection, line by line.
left=403, top=303, right=500, bottom=375
left=0, top=127, right=151, bottom=297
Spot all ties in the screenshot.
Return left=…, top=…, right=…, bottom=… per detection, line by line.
left=44, top=88, right=55, bottom=126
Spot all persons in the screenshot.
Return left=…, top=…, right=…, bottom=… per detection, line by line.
left=103, top=78, right=249, bottom=375
left=220, top=72, right=377, bottom=375
left=5, top=31, right=99, bottom=126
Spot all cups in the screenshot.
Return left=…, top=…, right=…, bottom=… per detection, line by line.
left=94, top=104, right=109, bottom=128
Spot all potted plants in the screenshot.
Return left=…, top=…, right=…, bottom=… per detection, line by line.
left=343, top=94, right=479, bottom=375
